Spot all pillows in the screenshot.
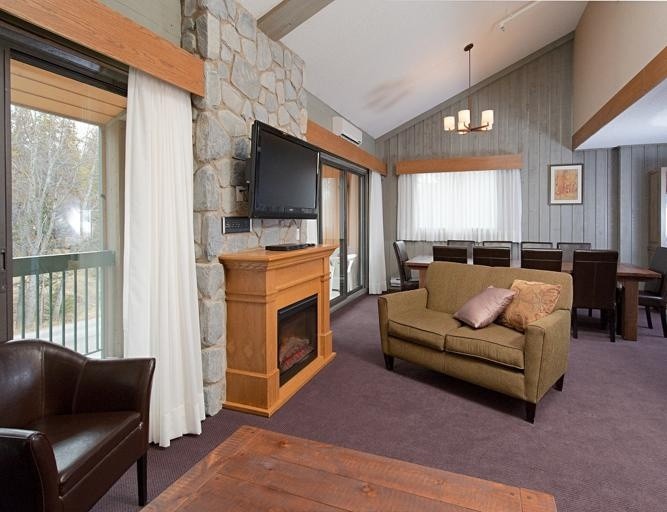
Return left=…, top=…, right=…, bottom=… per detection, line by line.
left=453, top=286, right=517, bottom=330
left=495, top=279, right=561, bottom=333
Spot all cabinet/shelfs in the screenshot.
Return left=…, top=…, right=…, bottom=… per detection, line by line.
left=648, top=166, right=666, bottom=267
left=218, top=243, right=340, bottom=418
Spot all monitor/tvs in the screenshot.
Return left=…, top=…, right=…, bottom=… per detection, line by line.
left=248, top=119, right=320, bottom=220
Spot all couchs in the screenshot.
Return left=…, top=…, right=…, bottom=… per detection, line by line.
left=0, top=339, right=157, bottom=512
left=333, top=254, right=358, bottom=293
left=378, top=261, right=573, bottom=423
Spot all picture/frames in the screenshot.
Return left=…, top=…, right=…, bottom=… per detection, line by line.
left=549, top=164, right=584, bottom=205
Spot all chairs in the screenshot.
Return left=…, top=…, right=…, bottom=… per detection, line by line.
left=521, top=241, right=552, bottom=258
left=394, top=240, right=420, bottom=291
left=521, top=248, right=564, bottom=272
left=615, top=273, right=667, bottom=338
left=473, top=246, right=511, bottom=268
left=447, top=239, right=475, bottom=258
left=484, top=241, right=513, bottom=261
left=433, top=246, right=468, bottom=264
left=570, top=248, right=619, bottom=342
left=557, top=242, right=591, bottom=262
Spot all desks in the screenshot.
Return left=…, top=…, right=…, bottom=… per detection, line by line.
left=405, top=254, right=660, bottom=342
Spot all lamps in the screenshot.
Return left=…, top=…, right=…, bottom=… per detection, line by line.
left=443, top=45, right=494, bottom=134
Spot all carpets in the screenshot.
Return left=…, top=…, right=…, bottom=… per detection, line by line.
left=141, top=425, right=557, bottom=512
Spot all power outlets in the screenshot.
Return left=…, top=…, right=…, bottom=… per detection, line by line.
left=236, top=185, right=245, bottom=202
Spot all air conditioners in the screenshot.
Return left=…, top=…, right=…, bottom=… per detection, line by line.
left=332, top=116, right=362, bottom=147
